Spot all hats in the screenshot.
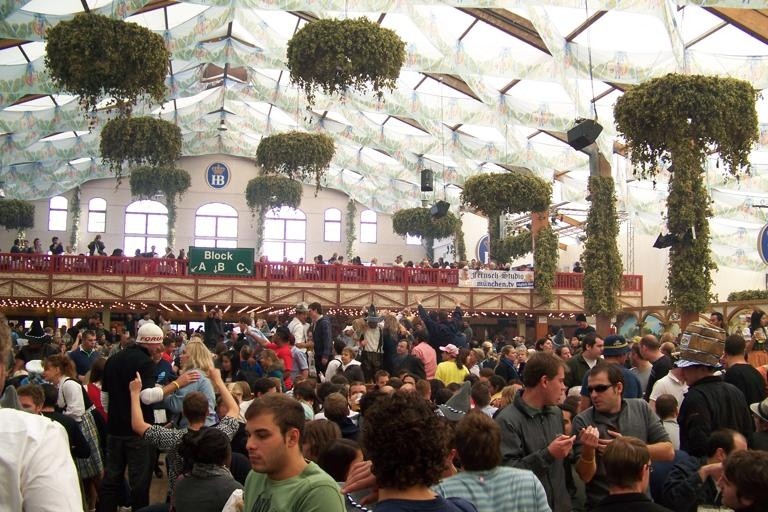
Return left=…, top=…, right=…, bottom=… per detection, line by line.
left=25, top=360, right=45, bottom=373
left=364, top=305, right=384, bottom=322
left=750, top=397, right=768, bottom=423
left=344, top=488, right=378, bottom=512
left=25, top=320, right=46, bottom=338
left=136, top=323, right=163, bottom=343
left=439, top=344, right=459, bottom=357
left=576, top=314, right=586, bottom=321
left=436, top=381, right=470, bottom=421
left=552, top=329, right=568, bottom=347
left=604, top=336, right=631, bottom=355
left=296, top=302, right=308, bottom=312
left=674, top=322, right=726, bottom=368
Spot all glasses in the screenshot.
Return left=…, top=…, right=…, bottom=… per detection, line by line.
left=647, top=465, right=655, bottom=472
left=588, top=384, right=613, bottom=392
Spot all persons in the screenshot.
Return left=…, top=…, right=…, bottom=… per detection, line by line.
left=0, top=297, right=767, bottom=511
left=574, top=260, right=585, bottom=271
left=2, top=233, right=535, bottom=282
